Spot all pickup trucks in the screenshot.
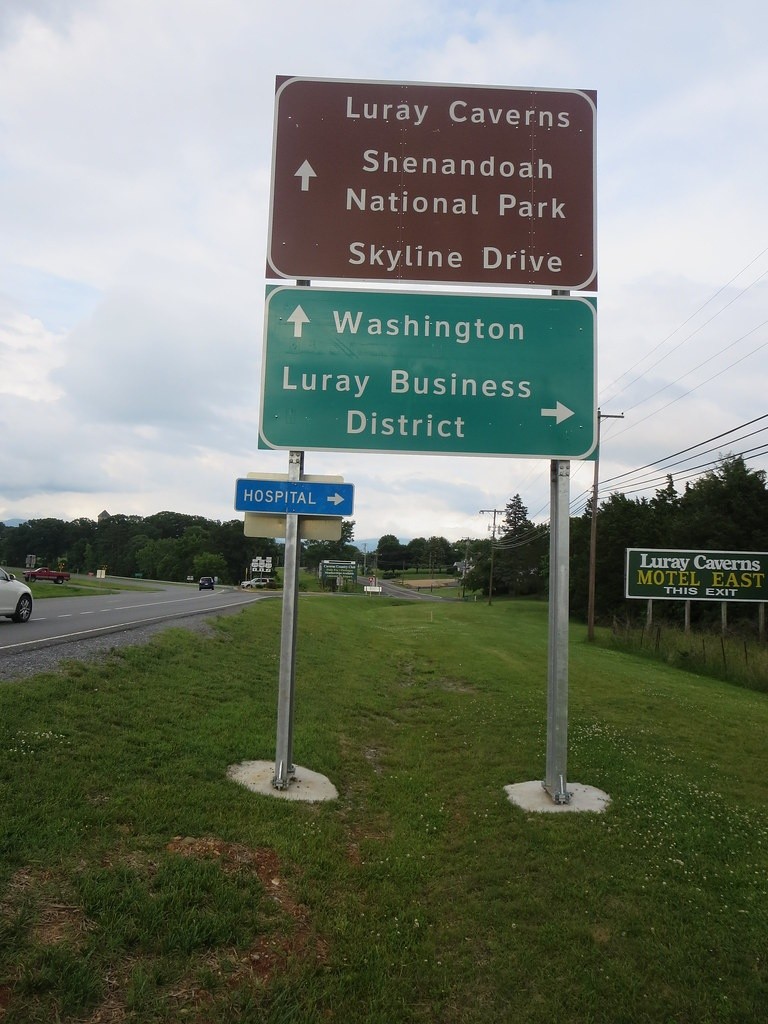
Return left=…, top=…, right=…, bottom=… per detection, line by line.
left=23, top=567, right=71, bottom=584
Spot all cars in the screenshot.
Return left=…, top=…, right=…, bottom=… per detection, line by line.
left=198, top=576, right=215, bottom=590
left=0, top=565, right=33, bottom=623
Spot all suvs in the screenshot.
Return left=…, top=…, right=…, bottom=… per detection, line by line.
left=241, top=578, right=270, bottom=587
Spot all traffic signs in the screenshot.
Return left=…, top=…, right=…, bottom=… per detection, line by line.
left=235, top=478, right=356, bottom=516
left=259, top=284, right=603, bottom=461
left=265, top=76, right=599, bottom=290
left=625, top=545, right=768, bottom=604
